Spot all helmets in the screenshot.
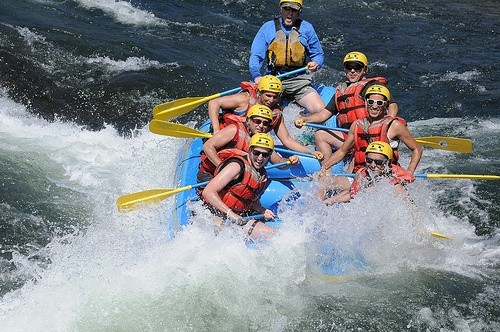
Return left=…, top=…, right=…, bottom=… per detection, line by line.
left=280, top=0, right=303, bottom=9
left=364, top=85, right=390, bottom=102
left=365, top=142, right=393, bottom=160
left=247, top=104, right=272, bottom=123
left=249, top=133, right=274, bottom=152
left=257, top=75, right=282, bottom=95
left=344, top=52, right=368, bottom=69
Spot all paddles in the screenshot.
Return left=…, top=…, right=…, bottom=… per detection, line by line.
left=149, top=119, right=319, bottom=159
left=116, top=159, right=290, bottom=214
left=317, top=175, right=500, bottom=179
left=152, top=64, right=311, bottom=120
left=300, top=122, right=472, bottom=152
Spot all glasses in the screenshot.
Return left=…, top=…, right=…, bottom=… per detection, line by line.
left=366, top=99, right=387, bottom=106
left=365, top=157, right=388, bottom=165
left=251, top=118, right=269, bottom=126
left=282, top=7, right=299, bottom=12
left=345, top=65, right=364, bottom=71
left=251, top=149, right=270, bottom=158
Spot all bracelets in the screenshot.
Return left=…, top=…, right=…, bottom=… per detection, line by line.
left=227, top=209, right=231, bottom=216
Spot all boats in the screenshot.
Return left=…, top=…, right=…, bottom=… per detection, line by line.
left=170, top=82, right=380, bottom=284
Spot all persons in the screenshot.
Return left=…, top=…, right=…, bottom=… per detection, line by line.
left=197, top=75, right=324, bottom=242
left=294, top=52, right=423, bottom=206
left=249, top=0, right=326, bottom=117
